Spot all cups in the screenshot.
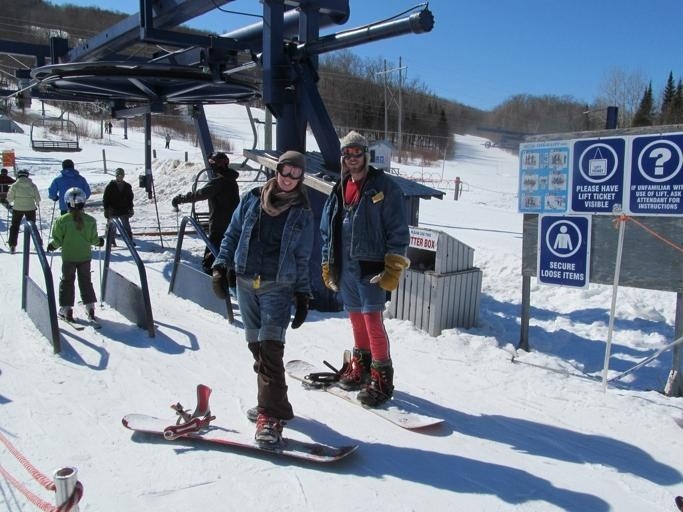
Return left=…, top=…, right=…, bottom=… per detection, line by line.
left=340, top=143, right=365, bottom=158
left=277, top=163, right=304, bottom=179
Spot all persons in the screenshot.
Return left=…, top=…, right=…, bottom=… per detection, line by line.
left=551, top=224, right=577, bottom=251
left=164, top=132, right=171, bottom=149
left=46, top=187, right=104, bottom=318
left=170, top=151, right=241, bottom=287
left=103, top=120, right=108, bottom=133
left=209, top=148, right=315, bottom=451
left=318, top=128, right=412, bottom=409
left=47, top=159, right=90, bottom=219
left=107, top=121, right=112, bottom=133
left=101, top=168, right=137, bottom=248
left=0, top=169, right=16, bottom=209
left=6, top=168, right=41, bottom=255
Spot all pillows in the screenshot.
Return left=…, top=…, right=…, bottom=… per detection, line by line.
left=17, top=170, right=28, bottom=177
left=63, top=187, right=87, bottom=206
left=208, top=152, right=230, bottom=169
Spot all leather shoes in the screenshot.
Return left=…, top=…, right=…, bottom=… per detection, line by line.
left=52, top=195, right=60, bottom=201
left=290, top=293, right=310, bottom=329
left=212, top=263, right=231, bottom=299
left=46, top=244, right=57, bottom=253
left=97, top=237, right=107, bottom=248
left=171, top=195, right=183, bottom=207
left=321, top=263, right=337, bottom=290
left=369, top=253, right=411, bottom=292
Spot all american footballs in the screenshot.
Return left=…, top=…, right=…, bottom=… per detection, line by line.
left=285, top=359, right=446, bottom=429
left=122, top=413, right=360, bottom=463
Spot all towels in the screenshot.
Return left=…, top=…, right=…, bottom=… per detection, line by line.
left=390, top=224, right=482, bottom=337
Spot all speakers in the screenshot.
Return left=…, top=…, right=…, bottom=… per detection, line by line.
left=246, top=385, right=295, bottom=424
left=85, top=302, right=96, bottom=322
left=338, top=346, right=373, bottom=391
left=255, top=410, right=286, bottom=450
left=356, top=360, right=395, bottom=407
left=7, top=243, right=17, bottom=254
left=57, top=303, right=75, bottom=321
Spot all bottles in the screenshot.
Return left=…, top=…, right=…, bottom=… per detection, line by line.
left=338, top=130, right=370, bottom=165
left=115, top=167, right=125, bottom=177
left=277, top=148, right=306, bottom=169
left=61, top=158, right=75, bottom=169
